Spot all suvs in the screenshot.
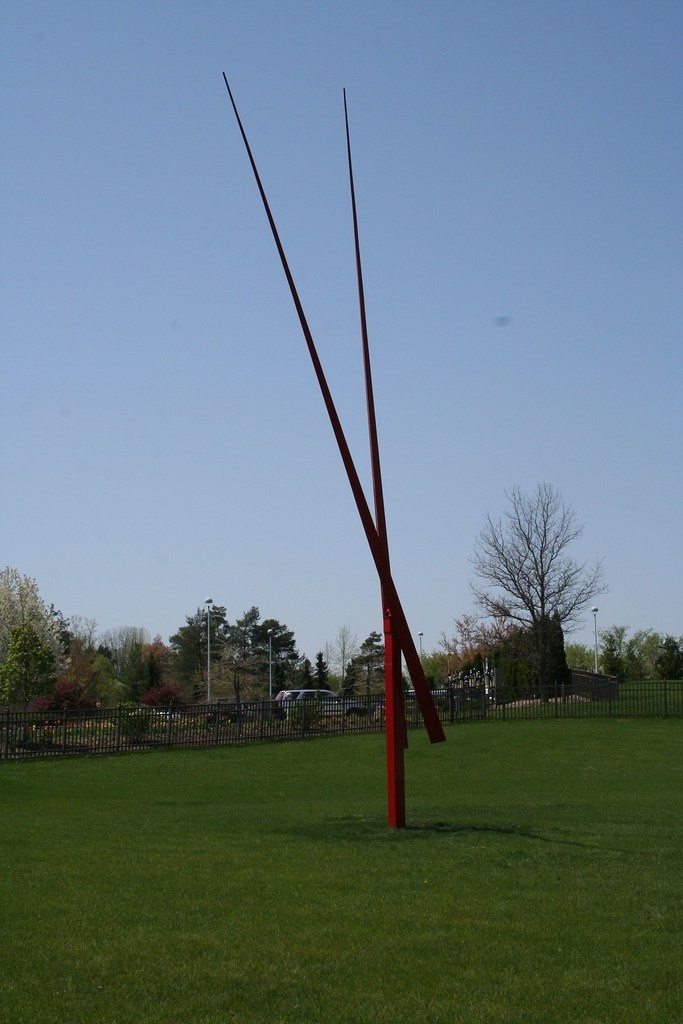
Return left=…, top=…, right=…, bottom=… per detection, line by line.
left=272, top=689, right=367, bottom=723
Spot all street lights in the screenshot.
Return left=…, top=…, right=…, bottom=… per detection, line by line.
left=204, top=596, right=213, bottom=700
left=267, top=628, right=275, bottom=699
left=418, top=631, right=424, bottom=663
left=592, top=607, right=599, bottom=673
